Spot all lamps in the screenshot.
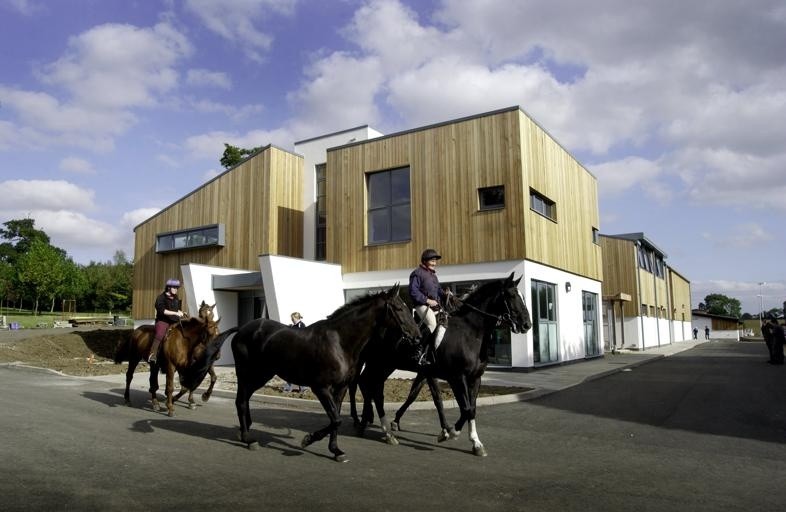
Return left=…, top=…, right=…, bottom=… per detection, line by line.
left=566, top=282, right=571, bottom=292
left=661, top=306, right=663, bottom=311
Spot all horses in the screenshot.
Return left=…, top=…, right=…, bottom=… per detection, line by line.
left=348, top=284, right=479, bottom=443
left=180, top=282, right=423, bottom=463
left=358, top=271, right=531, bottom=457
left=115, top=300, right=221, bottom=417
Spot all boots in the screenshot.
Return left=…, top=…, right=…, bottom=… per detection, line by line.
left=146, top=338, right=161, bottom=365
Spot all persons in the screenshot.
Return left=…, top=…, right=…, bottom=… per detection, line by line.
left=761, top=318, right=775, bottom=363
left=408, top=249, right=452, bottom=364
left=693, top=327, right=698, bottom=339
left=147, top=279, right=188, bottom=362
left=770, top=317, right=786, bottom=362
left=283, top=313, right=306, bottom=394
left=705, top=325, right=710, bottom=340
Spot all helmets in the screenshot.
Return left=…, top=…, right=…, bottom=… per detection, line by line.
left=421, top=249, right=442, bottom=263
left=166, top=278, right=182, bottom=288
